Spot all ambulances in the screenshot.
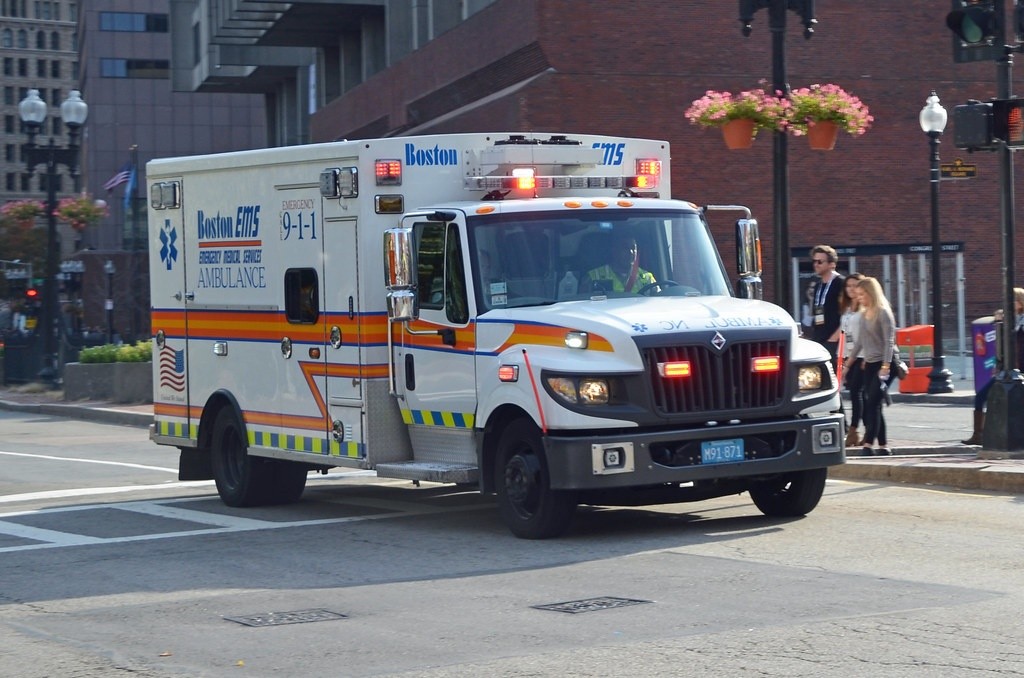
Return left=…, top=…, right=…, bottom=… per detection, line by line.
left=142, top=133, right=848, bottom=539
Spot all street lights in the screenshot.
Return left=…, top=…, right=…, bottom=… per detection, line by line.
left=734, top=1, right=819, bottom=314
left=919, top=89, right=955, bottom=395
left=102, top=259, right=116, bottom=346
left=17, top=87, right=90, bottom=394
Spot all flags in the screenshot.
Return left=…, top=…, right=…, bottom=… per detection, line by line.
left=102, top=161, right=136, bottom=205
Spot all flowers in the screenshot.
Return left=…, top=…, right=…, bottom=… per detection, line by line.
left=0, top=191, right=110, bottom=229
left=685, top=77, right=876, bottom=141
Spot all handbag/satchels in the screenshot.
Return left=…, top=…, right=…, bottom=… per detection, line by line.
left=892, top=359, right=909, bottom=380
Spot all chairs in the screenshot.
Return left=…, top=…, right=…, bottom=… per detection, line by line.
left=453, top=231, right=611, bottom=297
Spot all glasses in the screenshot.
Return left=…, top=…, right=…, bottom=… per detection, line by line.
left=812, top=259, right=832, bottom=264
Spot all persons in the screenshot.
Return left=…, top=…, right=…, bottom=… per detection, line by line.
left=802, top=278, right=817, bottom=327
left=583, top=232, right=660, bottom=294
left=961, top=288, right=1024, bottom=445
left=805, top=245, right=897, bottom=448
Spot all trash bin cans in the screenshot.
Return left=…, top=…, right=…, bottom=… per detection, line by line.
left=896, top=324, right=935, bottom=394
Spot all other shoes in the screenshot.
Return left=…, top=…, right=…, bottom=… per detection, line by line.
left=877, top=448, right=891, bottom=455
left=862, top=447, right=875, bottom=456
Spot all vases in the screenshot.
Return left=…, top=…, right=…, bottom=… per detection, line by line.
left=19, top=217, right=34, bottom=226
left=804, top=120, right=838, bottom=152
left=73, top=222, right=87, bottom=230
left=721, top=117, right=756, bottom=149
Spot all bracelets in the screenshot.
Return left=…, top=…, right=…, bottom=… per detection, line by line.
left=845, top=365, right=850, bottom=368
left=881, top=365, right=890, bottom=369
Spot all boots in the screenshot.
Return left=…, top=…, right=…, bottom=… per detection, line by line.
left=961, top=410, right=987, bottom=445
left=858, top=426, right=867, bottom=446
left=846, top=426, right=858, bottom=447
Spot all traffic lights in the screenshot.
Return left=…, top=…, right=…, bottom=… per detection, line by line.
left=25, top=287, right=42, bottom=300
left=992, top=97, right=1024, bottom=151
left=945, top=0, right=1008, bottom=65
